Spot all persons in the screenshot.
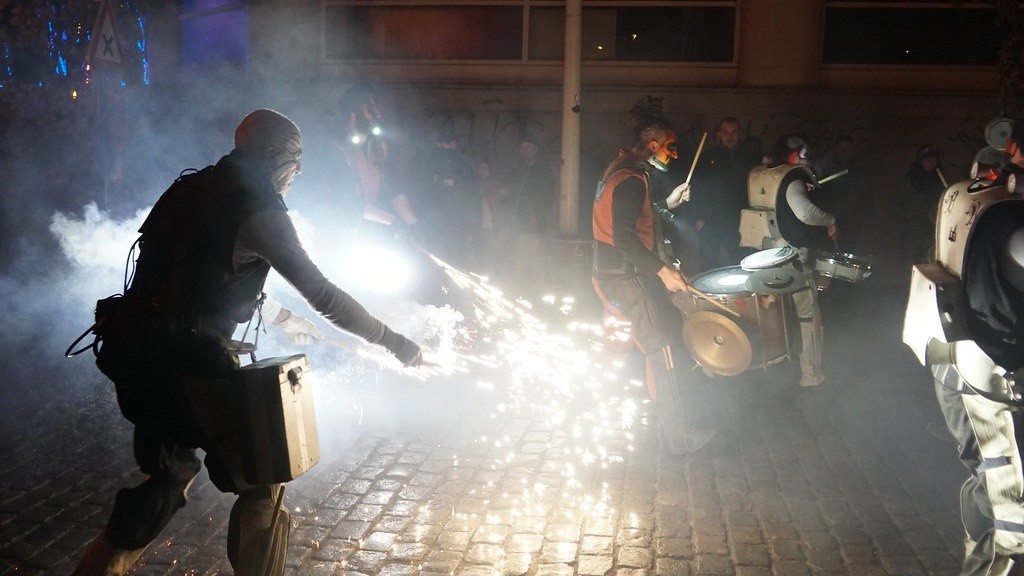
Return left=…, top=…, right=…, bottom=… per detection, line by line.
left=690, top=117, right=751, bottom=278
left=70, top=106, right=428, bottom=576
left=736, top=130, right=848, bottom=392
left=315, top=86, right=558, bottom=331
left=923, top=120, right=1024, bottom=576
left=581, top=111, right=703, bottom=502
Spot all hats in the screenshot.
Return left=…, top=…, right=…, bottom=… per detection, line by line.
left=915, top=144, right=938, bottom=159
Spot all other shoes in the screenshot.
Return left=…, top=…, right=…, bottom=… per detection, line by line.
left=799, top=375, right=827, bottom=387
left=669, top=427, right=717, bottom=457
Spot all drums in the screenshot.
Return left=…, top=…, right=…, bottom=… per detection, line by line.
left=739, top=247, right=807, bottom=295
left=688, top=264, right=798, bottom=376
left=813, top=246, right=874, bottom=285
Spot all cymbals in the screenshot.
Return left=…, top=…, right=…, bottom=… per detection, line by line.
left=682, top=310, right=752, bottom=377
left=745, top=267, right=806, bottom=295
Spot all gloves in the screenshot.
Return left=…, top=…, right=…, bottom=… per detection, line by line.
left=278, top=308, right=322, bottom=347
left=394, top=335, right=424, bottom=368
